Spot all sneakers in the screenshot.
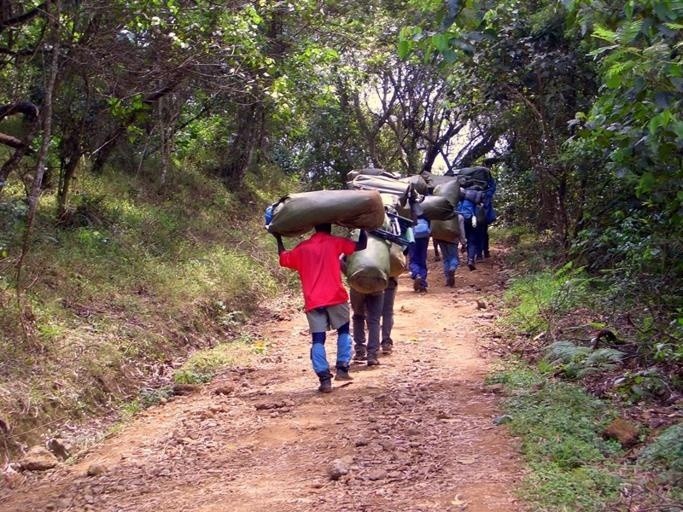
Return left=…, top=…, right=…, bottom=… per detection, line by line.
left=382, top=343, right=392, bottom=354
left=467, top=252, right=490, bottom=270
left=354, top=352, right=365, bottom=360
left=368, top=359, right=379, bottom=366
left=414, top=274, right=428, bottom=293
left=445, top=268, right=455, bottom=287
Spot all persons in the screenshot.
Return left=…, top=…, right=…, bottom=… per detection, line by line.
left=265, top=220, right=368, bottom=394
left=337, top=228, right=385, bottom=366
left=402, top=174, right=497, bottom=294
left=363, top=219, right=407, bottom=355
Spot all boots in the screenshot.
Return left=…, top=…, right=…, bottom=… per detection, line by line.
left=318, top=370, right=331, bottom=393
left=334, top=361, right=353, bottom=380
